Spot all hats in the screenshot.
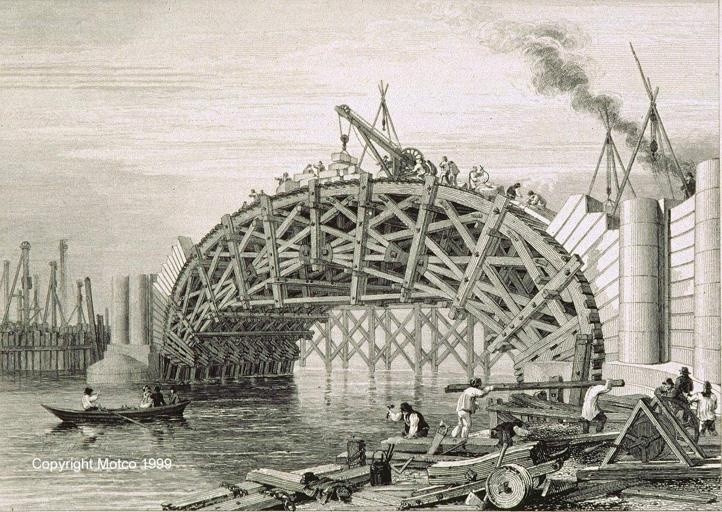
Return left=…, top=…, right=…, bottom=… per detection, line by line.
left=300, top=472, right=318, bottom=484
left=401, top=403, right=412, bottom=410
left=686, top=172, right=694, bottom=178
left=704, top=381, right=712, bottom=389
left=662, top=378, right=674, bottom=386
left=679, top=366, right=691, bottom=374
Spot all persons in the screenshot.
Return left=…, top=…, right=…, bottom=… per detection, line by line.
left=452, top=378, right=495, bottom=439
left=491, top=418, right=529, bottom=444
left=581, top=375, right=611, bottom=433
left=137, top=382, right=179, bottom=408
left=374, top=153, right=393, bottom=175
left=676, top=172, right=695, bottom=200
left=412, top=154, right=482, bottom=193
left=652, top=368, right=719, bottom=437
left=527, top=190, right=545, bottom=208
left=245, top=161, right=327, bottom=202
left=82, top=386, right=100, bottom=411
left=384, top=403, right=429, bottom=441
left=507, top=181, right=520, bottom=200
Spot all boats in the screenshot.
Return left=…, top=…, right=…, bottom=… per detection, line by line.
left=42, top=398, right=191, bottom=423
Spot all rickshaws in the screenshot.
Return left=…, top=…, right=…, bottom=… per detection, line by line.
left=397, top=440, right=570, bottom=511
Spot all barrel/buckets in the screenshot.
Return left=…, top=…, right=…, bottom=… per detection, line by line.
left=370, top=449, right=390, bottom=486
